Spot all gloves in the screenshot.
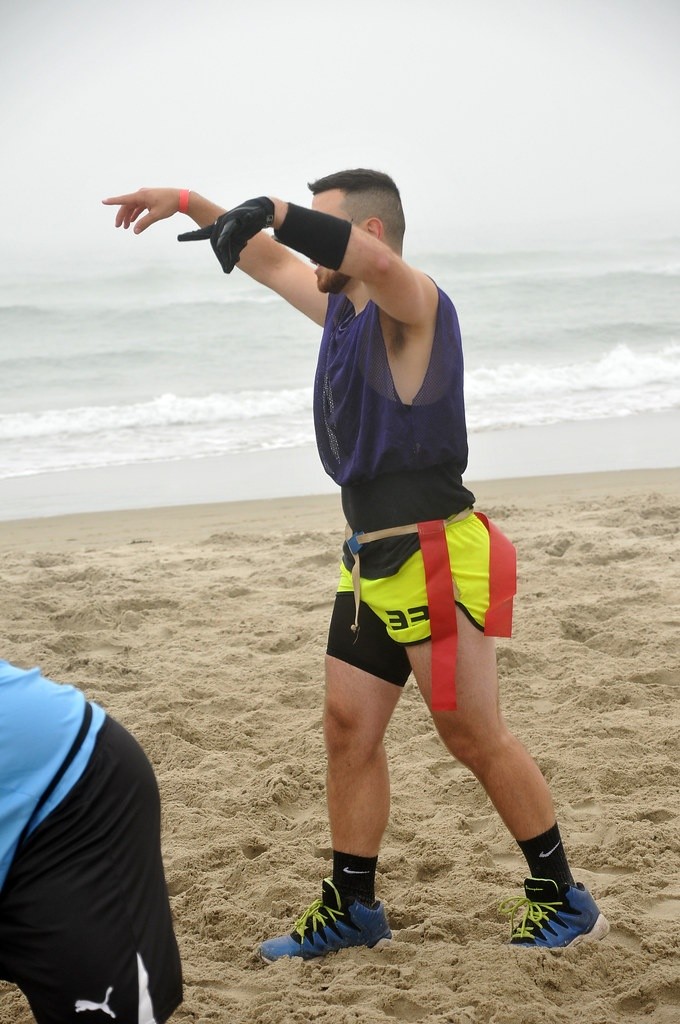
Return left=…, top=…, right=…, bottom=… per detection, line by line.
left=177, top=197, right=275, bottom=274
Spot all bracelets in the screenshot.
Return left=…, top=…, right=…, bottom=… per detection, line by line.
left=179, top=188, right=191, bottom=213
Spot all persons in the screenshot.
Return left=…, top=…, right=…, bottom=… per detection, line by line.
left=101, top=169, right=610, bottom=962
left=0, top=660, right=183, bottom=1024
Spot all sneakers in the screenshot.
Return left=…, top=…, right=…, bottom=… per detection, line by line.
left=259, top=878, right=392, bottom=965
left=499, top=878, right=611, bottom=948
